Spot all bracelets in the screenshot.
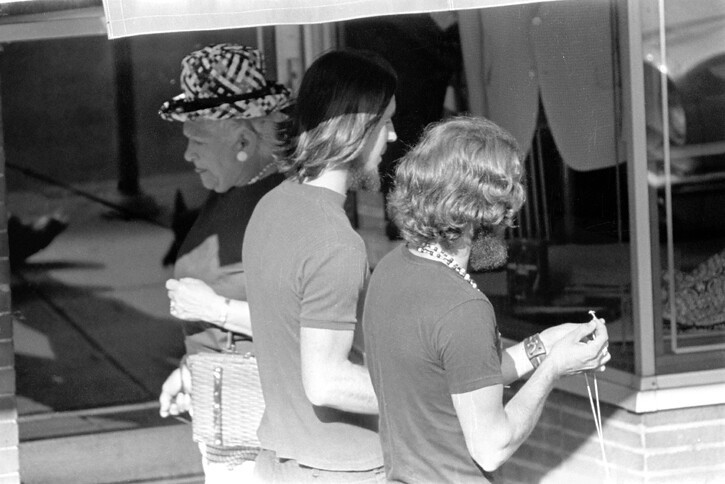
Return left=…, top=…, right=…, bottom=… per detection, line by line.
left=219, top=298, right=230, bottom=328
left=524, top=333, right=546, bottom=369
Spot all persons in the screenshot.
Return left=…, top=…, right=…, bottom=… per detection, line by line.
left=241, top=50, right=399, bottom=484
left=362, top=116, right=611, bottom=484
left=158, top=43, right=294, bottom=484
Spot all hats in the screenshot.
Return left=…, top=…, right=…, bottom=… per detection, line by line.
left=157, top=43, right=291, bottom=122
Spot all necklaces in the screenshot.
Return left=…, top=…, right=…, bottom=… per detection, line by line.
left=416, top=242, right=480, bottom=291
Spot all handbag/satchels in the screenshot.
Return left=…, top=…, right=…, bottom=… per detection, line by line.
left=185, top=331, right=265, bottom=449
